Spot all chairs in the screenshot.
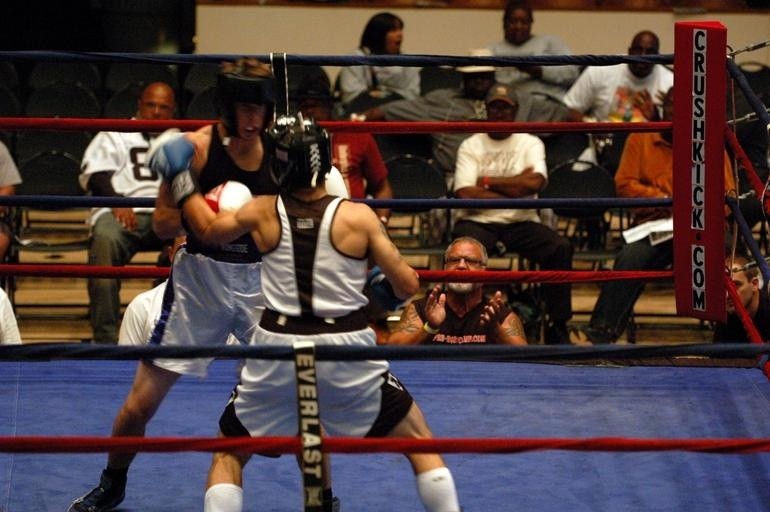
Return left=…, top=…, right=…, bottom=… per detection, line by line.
left=361, top=151, right=449, bottom=310
left=537, top=157, right=636, bottom=344
left=7, top=148, right=93, bottom=313
left=727, top=60, right=770, bottom=171
left=419, top=65, right=461, bottom=98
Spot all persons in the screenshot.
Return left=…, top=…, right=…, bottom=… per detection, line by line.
left=144, top=113, right=461, bottom=512
left=78, top=81, right=181, bottom=348
left=66, top=57, right=281, bottom=512
left=271, top=0, right=769, bottom=370
left=0, top=141, right=25, bottom=346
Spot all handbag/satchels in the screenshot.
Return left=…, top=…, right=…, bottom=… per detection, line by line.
left=331, top=74, right=404, bottom=118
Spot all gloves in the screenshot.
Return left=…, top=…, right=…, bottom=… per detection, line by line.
left=145, top=127, right=200, bottom=207
left=369, top=264, right=412, bottom=310
left=205, top=180, right=253, bottom=215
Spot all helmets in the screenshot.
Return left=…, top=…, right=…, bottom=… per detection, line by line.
left=266, top=114, right=333, bottom=190
left=215, top=61, right=275, bottom=137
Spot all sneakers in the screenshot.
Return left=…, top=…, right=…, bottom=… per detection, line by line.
left=542, top=317, right=573, bottom=346
left=576, top=322, right=614, bottom=348
left=67, top=471, right=129, bottom=511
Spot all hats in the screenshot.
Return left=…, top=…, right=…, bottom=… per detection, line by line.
left=290, top=66, right=343, bottom=103
left=456, top=48, right=496, bottom=74
left=486, top=84, right=519, bottom=109
